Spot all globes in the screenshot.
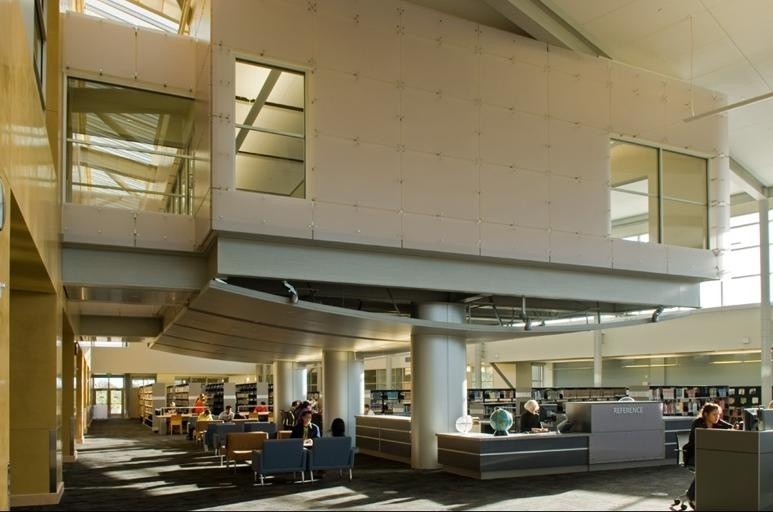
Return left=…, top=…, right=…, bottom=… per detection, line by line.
left=489, top=409, right=512, bottom=435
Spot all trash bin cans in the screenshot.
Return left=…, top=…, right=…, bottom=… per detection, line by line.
left=156, top=416, right=168, bottom=434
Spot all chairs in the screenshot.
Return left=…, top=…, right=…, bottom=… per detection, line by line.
left=164, top=412, right=361, bottom=487
left=670, top=429, right=695, bottom=511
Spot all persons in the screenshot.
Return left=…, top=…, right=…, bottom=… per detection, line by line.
left=314, top=416, right=346, bottom=479
left=289, top=410, right=322, bottom=480
left=519, top=399, right=549, bottom=434
left=190, top=393, right=207, bottom=416
left=197, top=409, right=216, bottom=423
left=282, top=391, right=320, bottom=432
left=363, top=404, right=375, bottom=415
left=680, top=401, right=734, bottom=512
left=231, top=413, right=248, bottom=420
left=184, top=408, right=197, bottom=441
left=217, top=406, right=234, bottom=424
left=253, top=401, right=269, bottom=423
left=162, top=401, right=177, bottom=415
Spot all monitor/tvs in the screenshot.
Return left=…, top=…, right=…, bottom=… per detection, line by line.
left=742, top=408, right=762, bottom=431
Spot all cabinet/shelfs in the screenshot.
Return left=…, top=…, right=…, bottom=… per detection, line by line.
left=651, top=384, right=762, bottom=425
left=137, top=383, right=273, bottom=433
